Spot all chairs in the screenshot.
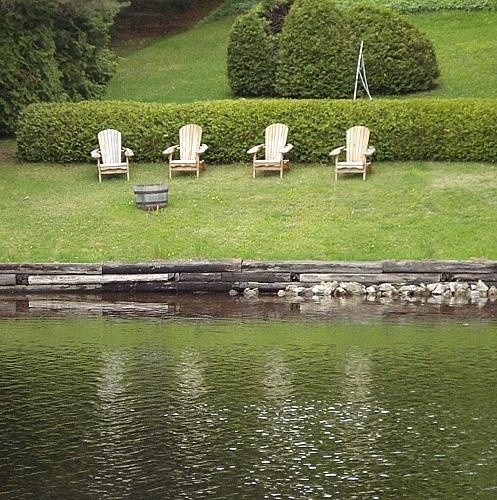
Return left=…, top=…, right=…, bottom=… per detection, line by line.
left=162, top=124, right=208, bottom=179
left=246, top=122, right=293, bottom=179
left=90, top=128, right=134, bottom=183
left=329, top=124, right=376, bottom=181
